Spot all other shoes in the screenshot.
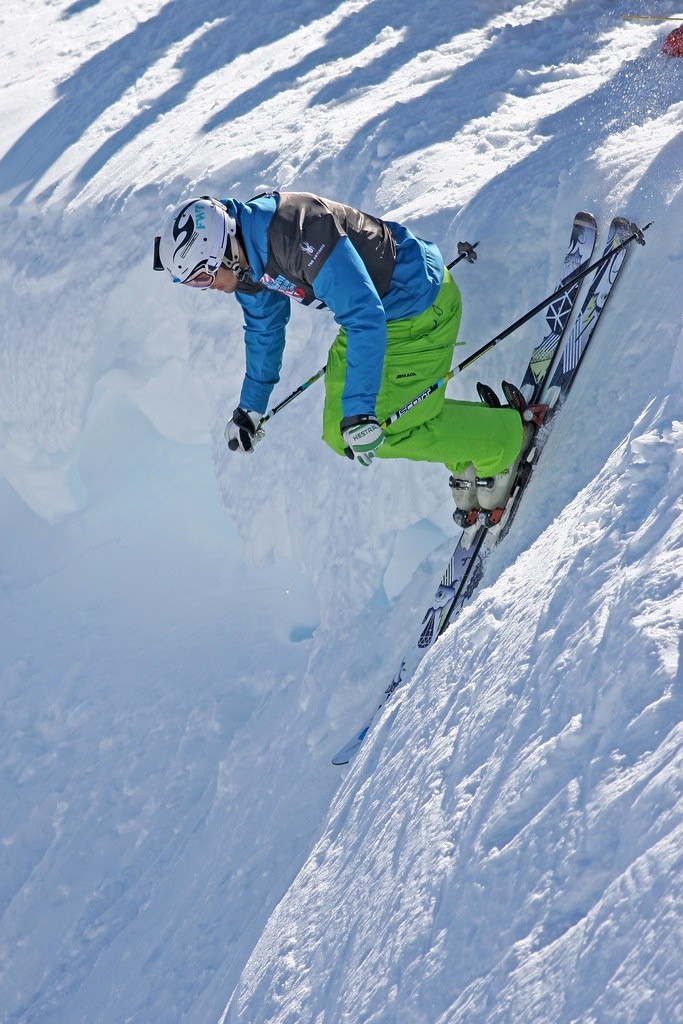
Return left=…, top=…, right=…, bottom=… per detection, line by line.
left=450, top=400, right=533, bottom=511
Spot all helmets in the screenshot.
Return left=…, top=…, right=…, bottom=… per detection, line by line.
left=158, top=198, right=236, bottom=291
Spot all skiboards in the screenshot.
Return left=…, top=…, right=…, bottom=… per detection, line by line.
left=329, top=211, right=645, bottom=767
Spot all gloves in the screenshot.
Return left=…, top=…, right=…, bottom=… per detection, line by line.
left=224, top=408, right=264, bottom=454
left=343, top=415, right=386, bottom=467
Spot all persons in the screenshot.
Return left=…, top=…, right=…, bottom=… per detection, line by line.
left=154, top=192, right=534, bottom=528
